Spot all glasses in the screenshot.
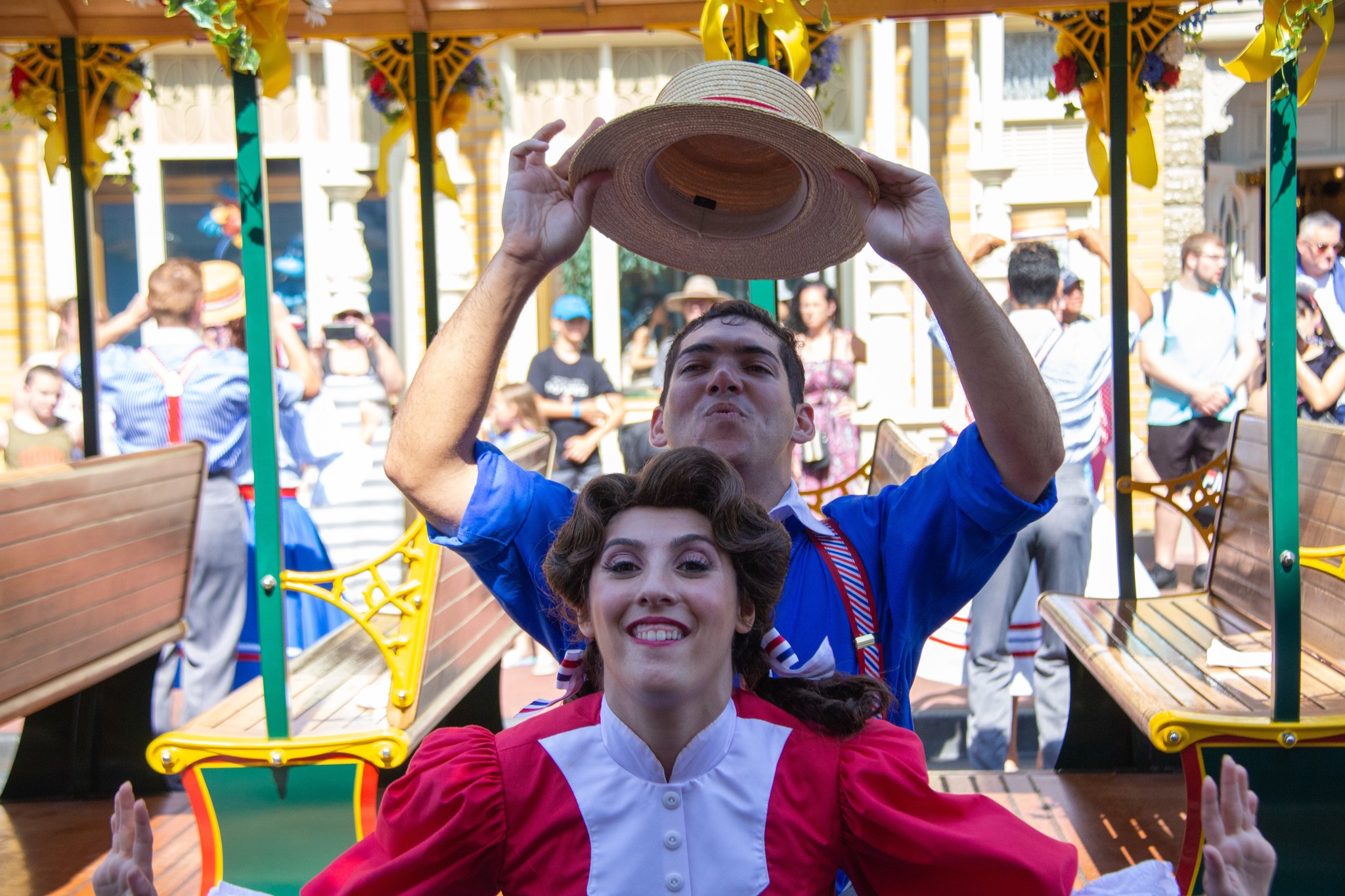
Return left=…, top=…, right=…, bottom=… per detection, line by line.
left=337, top=312, right=363, bottom=320
left=1305, top=241, right=1343, bottom=256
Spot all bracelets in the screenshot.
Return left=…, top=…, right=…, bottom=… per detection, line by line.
left=1225, top=385, right=1233, bottom=399
left=574, top=401, right=580, bottom=419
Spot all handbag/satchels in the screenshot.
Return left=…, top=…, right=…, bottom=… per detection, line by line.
left=800, top=427, right=830, bottom=473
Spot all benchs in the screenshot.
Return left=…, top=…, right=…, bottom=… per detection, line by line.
left=140, top=427, right=562, bottom=896
left=1027, top=401, right=1345, bottom=896
left=0, top=437, right=211, bottom=815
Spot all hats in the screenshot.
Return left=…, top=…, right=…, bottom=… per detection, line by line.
left=567, top=58, right=880, bottom=280
left=552, top=293, right=593, bottom=323
left=1060, top=266, right=1085, bottom=293
left=329, top=289, right=369, bottom=318
left=665, top=275, right=734, bottom=310
left=197, top=258, right=247, bottom=329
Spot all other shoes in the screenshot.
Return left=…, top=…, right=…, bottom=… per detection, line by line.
left=1147, top=562, right=1178, bottom=591
left=1192, top=563, right=1208, bottom=590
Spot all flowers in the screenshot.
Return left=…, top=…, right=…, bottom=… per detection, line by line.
left=348, top=39, right=504, bottom=211
left=10, top=37, right=159, bottom=204
left=783, top=18, right=846, bottom=91
left=1043, top=18, right=1188, bottom=199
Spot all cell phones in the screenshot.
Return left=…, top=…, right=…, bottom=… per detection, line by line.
left=324, top=326, right=356, bottom=340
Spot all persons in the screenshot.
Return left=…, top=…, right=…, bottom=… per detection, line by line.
left=486, top=383, right=545, bottom=452
left=170, top=318, right=354, bottom=695
left=0, top=364, right=120, bottom=470
left=1139, top=232, right=1260, bottom=589
left=294, top=291, right=407, bottom=615
left=924, top=226, right=1154, bottom=772
left=382, top=114, right=1066, bottom=894
left=1244, top=210, right=1345, bottom=426
left=18, top=295, right=113, bottom=380
left=92, top=449, right=1275, bottom=895
left=649, top=273, right=734, bottom=389
left=524, top=293, right=626, bottom=494
left=789, top=278, right=864, bottom=519
left=58, top=256, right=322, bottom=734
left=620, top=294, right=669, bottom=386
left=1061, top=272, right=1090, bottom=324
